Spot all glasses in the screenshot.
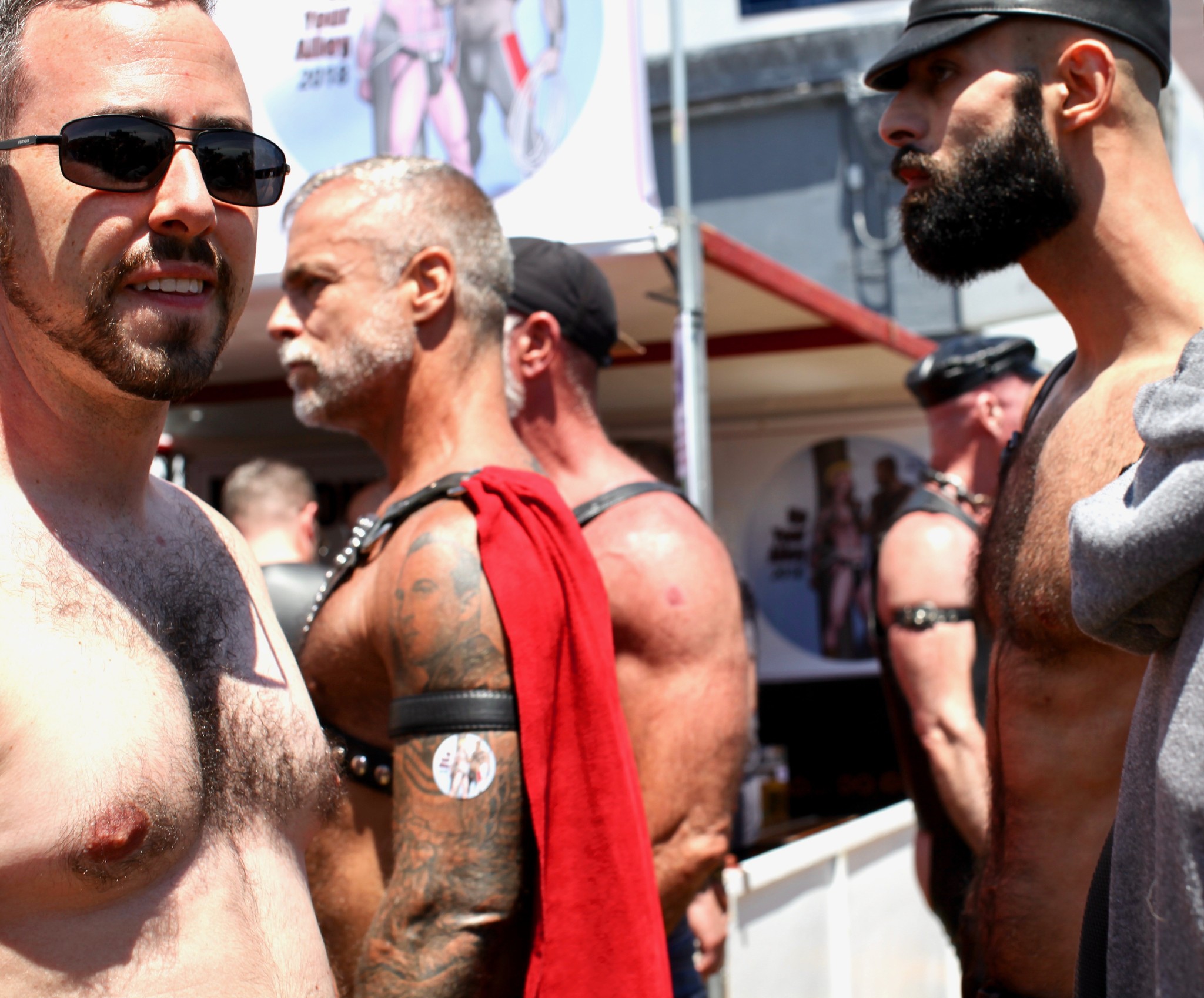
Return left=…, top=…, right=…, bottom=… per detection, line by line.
left=1, top=116, right=292, bottom=208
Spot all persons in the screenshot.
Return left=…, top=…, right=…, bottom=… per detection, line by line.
left=224, top=459, right=356, bottom=655
left=0, top=0, right=386, bottom=997
left=450, top=0, right=564, bottom=183
left=273, top=154, right=584, bottom=998
left=857, top=0, right=1204, bottom=997
left=356, top=0, right=477, bottom=182
left=351, top=232, right=762, bottom=998
left=868, top=331, right=1055, bottom=997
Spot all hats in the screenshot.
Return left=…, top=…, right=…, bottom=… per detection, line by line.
left=905, top=335, right=1046, bottom=410
left=504, top=237, right=618, bottom=369
left=865, top=0, right=1173, bottom=94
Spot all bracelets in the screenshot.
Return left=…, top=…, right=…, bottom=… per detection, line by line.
left=357, top=68, right=371, bottom=80
